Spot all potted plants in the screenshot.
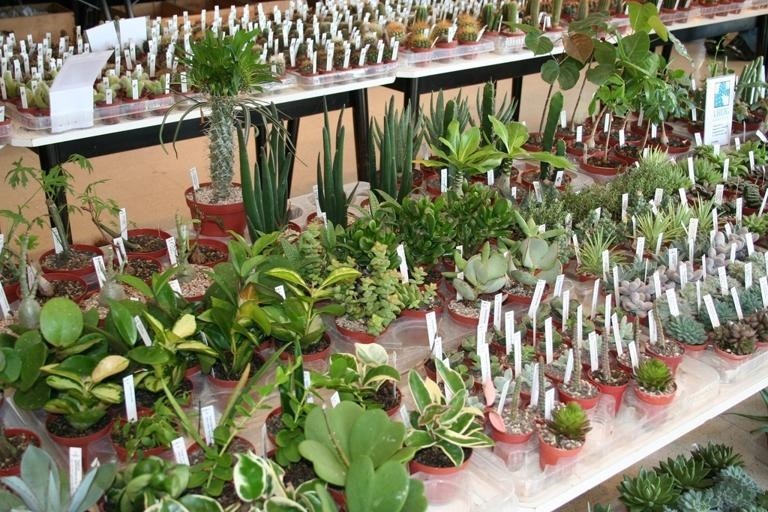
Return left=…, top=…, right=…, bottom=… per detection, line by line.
left=1, top=0, right=768, bottom=512
